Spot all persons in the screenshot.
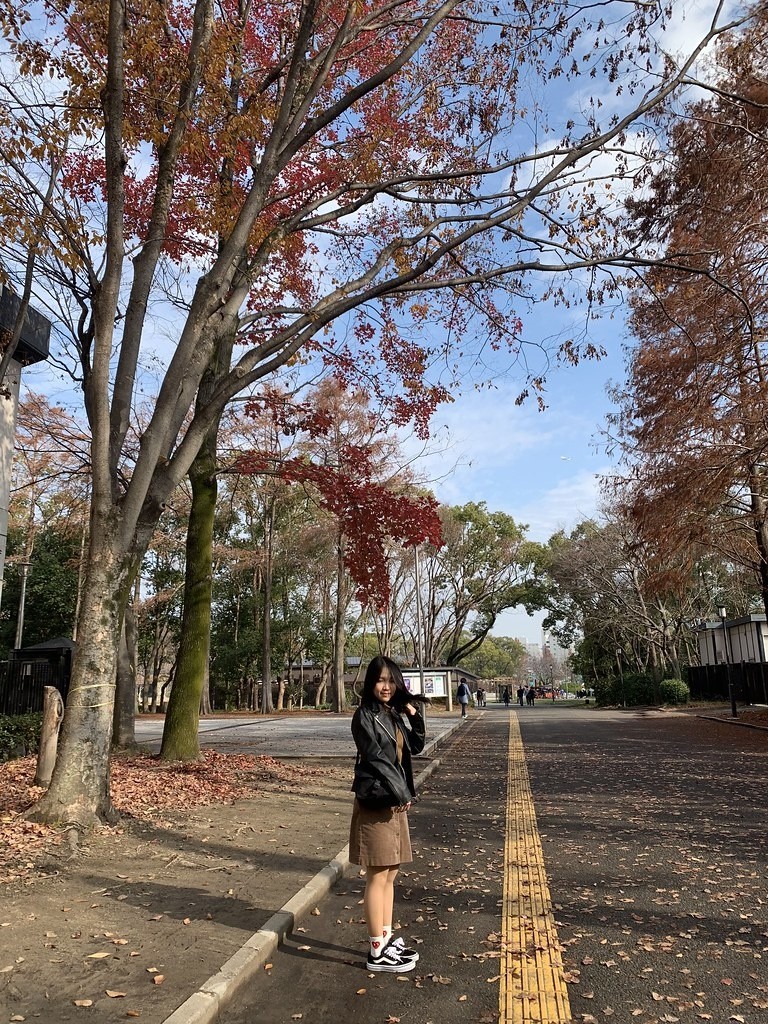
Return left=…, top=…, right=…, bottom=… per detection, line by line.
left=517, top=685, right=537, bottom=707
left=576, top=690, right=590, bottom=698
left=349, top=655, right=425, bottom=973
left=503, top=690, right=512, bottom=707
left=456, top=678, right=473, bottom=718
left=477, top=688, right=486, bottom=707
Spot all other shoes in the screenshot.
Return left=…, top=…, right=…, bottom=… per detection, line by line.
left=505, top=705, right=506, bottom=706
left=507, top=705, right=508, bottom=706
left=483, top=705, right=486, bottom=707
left=530, top=706, right=531, bottom=706
left=533, top=706, right=534, bottom=707
left=462, top=715, right=466, bottom=718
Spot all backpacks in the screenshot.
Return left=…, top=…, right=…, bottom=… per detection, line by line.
left=477, top=691, right=481, bottom=696
left=458, top=684, right=466, bottom=696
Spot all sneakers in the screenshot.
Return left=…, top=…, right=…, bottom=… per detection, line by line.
left=387, top=938, right=420, bottom=961
left=366, top=947, right=415, bottom=973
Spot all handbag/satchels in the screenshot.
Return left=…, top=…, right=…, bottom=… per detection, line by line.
left=351, top=762, right=407, bottom=809
